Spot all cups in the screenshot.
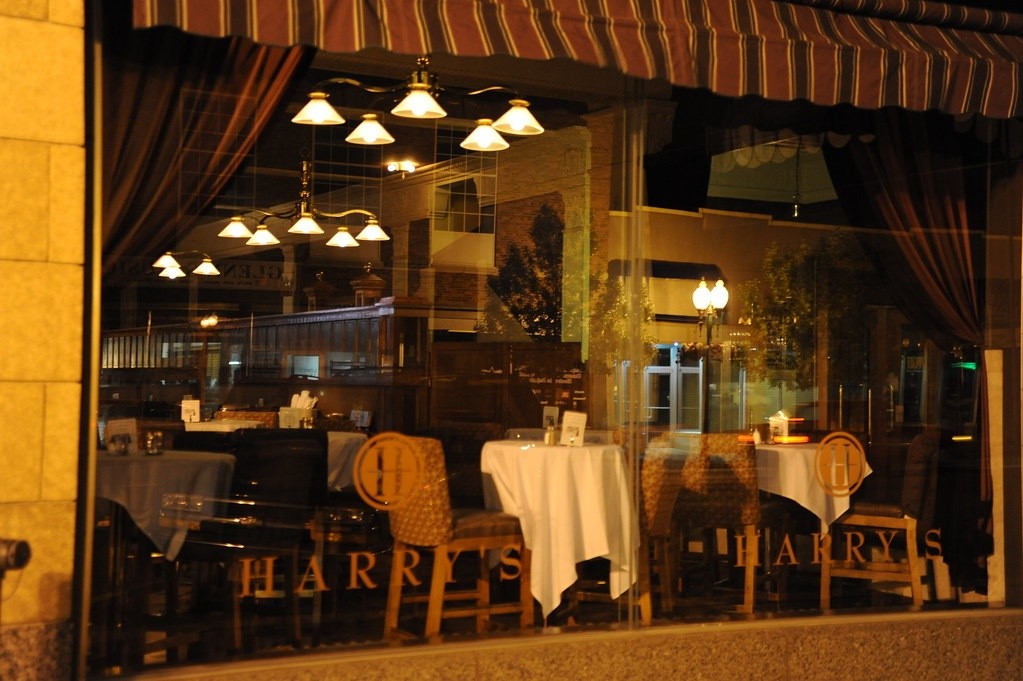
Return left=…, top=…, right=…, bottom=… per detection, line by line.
left=146, top=431, right=163, bottom=455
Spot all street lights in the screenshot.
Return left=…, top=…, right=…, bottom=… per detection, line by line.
left=691, top=277, right=728, bottom=434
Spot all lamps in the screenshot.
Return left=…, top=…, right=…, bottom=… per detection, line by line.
left=217, top=160, right=390, bottom=248
left=152, top=248, right=221, bottom=279
left=291, top=56, right=544, bottom=151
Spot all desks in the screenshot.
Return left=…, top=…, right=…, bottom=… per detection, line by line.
left=751, top=442, right=874, bottom=610
left=480, top=439, right=642, bottom=626
left=326, top=431, right=369, bottom=493
left=505, top=429, right=613, bottom=443
left=95, top=451, right=237, bottom=630
left=183, top=416, right=266, bottom=434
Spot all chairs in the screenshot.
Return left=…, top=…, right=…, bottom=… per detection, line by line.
left=125, top=410, right=946, bottom=670
